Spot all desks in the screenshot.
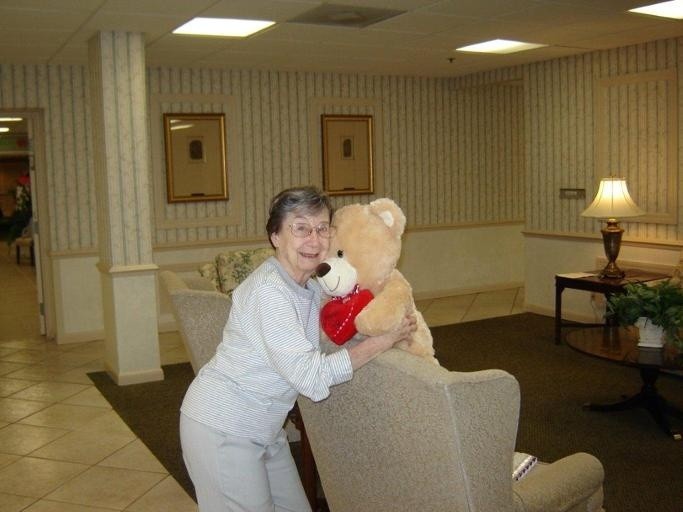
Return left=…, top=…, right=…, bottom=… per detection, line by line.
left=553, top=266, right=672, bottom=349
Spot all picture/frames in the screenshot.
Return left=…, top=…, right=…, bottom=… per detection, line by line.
left=319, top=112, right=376, bottom=197
left=162, top=111, right=229, bottom=205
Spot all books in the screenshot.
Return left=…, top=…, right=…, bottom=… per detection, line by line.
left=512, top=451, right=537, bottom=482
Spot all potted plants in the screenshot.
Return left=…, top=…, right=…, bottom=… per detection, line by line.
left=603, top=278, right=683, bottom=349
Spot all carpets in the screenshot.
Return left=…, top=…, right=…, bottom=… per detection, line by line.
left=84, top=308, right=681, bottom=512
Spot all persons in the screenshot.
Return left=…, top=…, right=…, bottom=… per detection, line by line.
left=178, top=186, right=416, bottom=512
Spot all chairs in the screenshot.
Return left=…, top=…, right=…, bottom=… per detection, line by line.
left=158, top=267, right=233, bottom=377
left=296, top=328, right=606, bottom=512
left=14, top=217, right=34, bottom=266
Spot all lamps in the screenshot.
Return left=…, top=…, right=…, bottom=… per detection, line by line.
left=583, top=177, right=647, bottom=277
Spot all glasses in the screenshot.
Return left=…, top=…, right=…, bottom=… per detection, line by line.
left=285, top=222, right=331, bottom=238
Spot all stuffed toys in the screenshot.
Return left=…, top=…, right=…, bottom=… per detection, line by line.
left=315, top=196, right=438, bottom=364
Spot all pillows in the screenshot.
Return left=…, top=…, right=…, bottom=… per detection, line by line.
left=199, top=246, right=273, bottom=298
